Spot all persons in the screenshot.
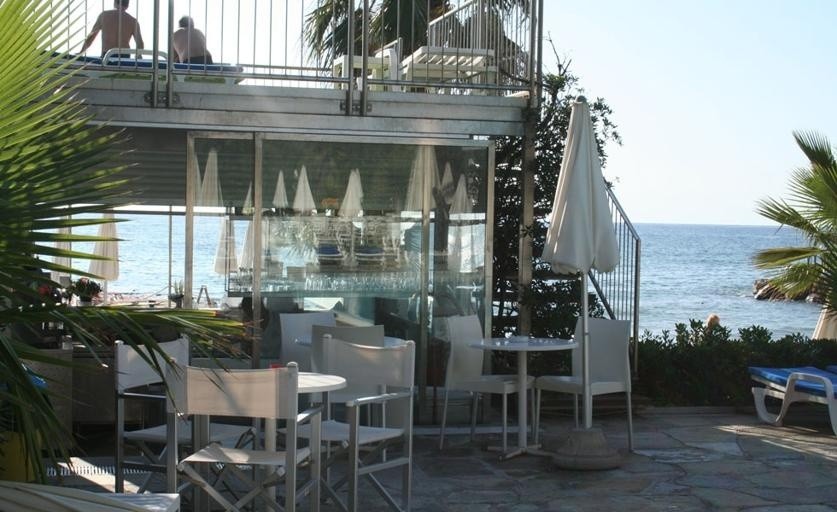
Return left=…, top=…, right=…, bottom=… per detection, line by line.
left=705, top=315, right=719, bottom=336
left=173, top=16, right=212, bottom=64
left=79, top=0, right=144, bottom=58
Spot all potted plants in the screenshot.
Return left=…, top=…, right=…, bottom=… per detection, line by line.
left=66, top=278, right=104, bottom=307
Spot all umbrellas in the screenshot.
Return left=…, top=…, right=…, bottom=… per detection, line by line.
left=540, top=96, right=620, bottom=430
left=87, top=208, right=120, bottom=298
left=189, top=137, right=474, bottom=228
left=214, top=212, right=269, bottom=295
left=49, top=229, right=68, bottom=285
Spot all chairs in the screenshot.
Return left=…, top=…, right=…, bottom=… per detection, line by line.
left=748, top=364, right=837, bottom=436
left=115, top=313, right=416, bottom=511
left=535, top=315, right=634, bottom=452
left=439, top=314, right=535, bottom=461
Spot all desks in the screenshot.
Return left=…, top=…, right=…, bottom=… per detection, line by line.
left=467, top=337, right=580, bottom=460
left=2, top=479, right=180, bottom=511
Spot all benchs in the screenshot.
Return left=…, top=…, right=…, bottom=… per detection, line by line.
left=39, top=47, right=245, bottom=84
left=355, top=38, right=497, bottom=97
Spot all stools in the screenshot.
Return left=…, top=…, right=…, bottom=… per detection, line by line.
left=332, top=48, right=401, bottom=92
